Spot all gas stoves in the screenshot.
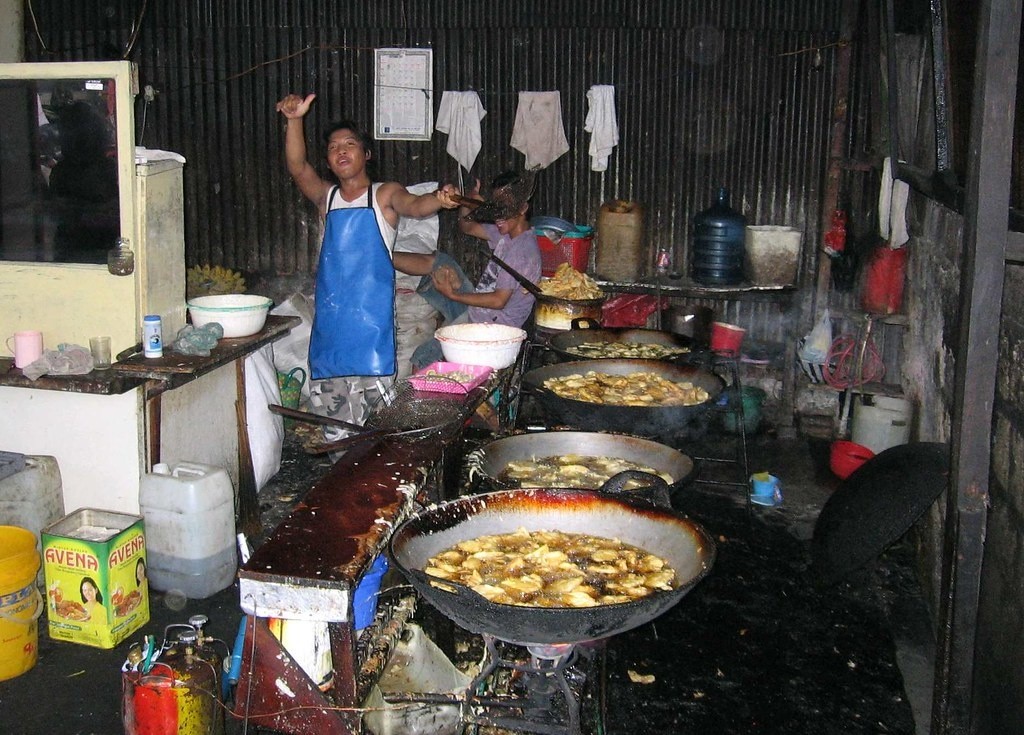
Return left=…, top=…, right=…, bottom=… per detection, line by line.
left=459, top=632, right=593, bottom=735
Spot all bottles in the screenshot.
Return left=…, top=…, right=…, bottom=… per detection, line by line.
left=143, top=314, right=163, bottom=358
left=750, top=473, right=780, bottom=506
left=692, top=187, right=746, bottom=285
left=107, top=238, right=134, bottom=276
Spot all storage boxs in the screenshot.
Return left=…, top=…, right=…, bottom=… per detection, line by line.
left=40, top=506, right=150, bottom=649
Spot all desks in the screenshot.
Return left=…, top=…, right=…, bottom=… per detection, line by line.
left=0, top=315, right=302, bottom=526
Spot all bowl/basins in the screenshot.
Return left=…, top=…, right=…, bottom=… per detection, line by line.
left=186, top=293, right=273, bottom=338
left=712, top=321, right=745, bottom=357
left=830, top=440, right=874, bottom=477
left=434, top=322, right=527, bottom=368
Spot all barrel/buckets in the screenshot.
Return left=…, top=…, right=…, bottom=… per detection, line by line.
left=139, top=460, right=238, bottom=599
left=723, top=384, right=766, bottom=433
left=595, top=199, right=644, bottom=284
left=747, top=225, right=798, bottom=286
left=851, top=396, right=911, bottom=455
left=1, top=526, right=42, bottom=681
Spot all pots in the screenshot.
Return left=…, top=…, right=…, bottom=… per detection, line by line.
left=487, top=252, right=608, bottom=306
left=520, top=359, right=727, bottom=435
left=550, top=318, right=710, bottom=364
left=472, top=431, right=696, bottom=499
left=389, top=470, right=717, bottom=645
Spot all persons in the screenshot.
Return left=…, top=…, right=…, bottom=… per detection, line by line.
left=275, top=92, right=461, bottom=463
left=392, top=171, right=542, bottom=376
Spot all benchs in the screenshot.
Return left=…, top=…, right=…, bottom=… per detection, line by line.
left=236, top=356, right=519, bottom=735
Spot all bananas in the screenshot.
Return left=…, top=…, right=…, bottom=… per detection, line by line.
left=185, top=262, right=247, bottom=299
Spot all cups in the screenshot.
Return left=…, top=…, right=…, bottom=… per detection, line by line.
left=90, top=337, right=112, bottom=370
left=5, top=331, right=44, bottom=368
